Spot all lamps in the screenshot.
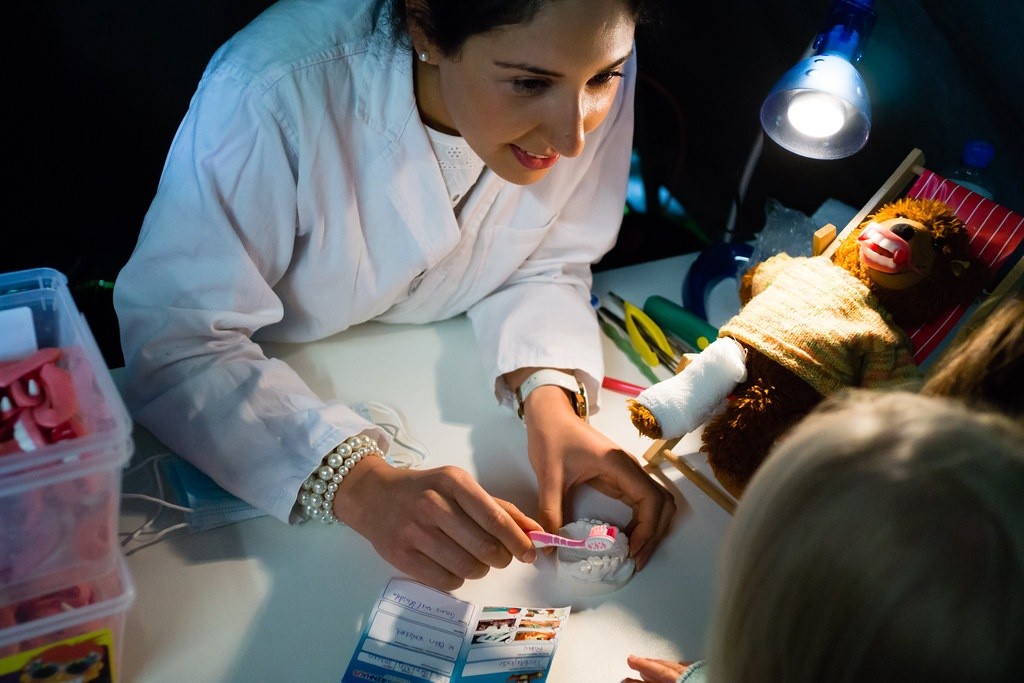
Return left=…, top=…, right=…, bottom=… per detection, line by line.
left=680, top=0, right=881, bottom=331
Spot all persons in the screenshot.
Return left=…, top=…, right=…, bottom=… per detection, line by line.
left=113, top=0, right=679, bottom=590
left=626, top=390, right=1024, bottom=683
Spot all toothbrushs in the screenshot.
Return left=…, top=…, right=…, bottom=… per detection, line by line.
left=524, top=525, right=617, bottom=552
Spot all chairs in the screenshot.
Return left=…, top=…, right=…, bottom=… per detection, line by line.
left=644, top=146, right=1024, bottom=516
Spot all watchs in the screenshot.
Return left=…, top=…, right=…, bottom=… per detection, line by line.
left=514, top=369, right=589, bottom=429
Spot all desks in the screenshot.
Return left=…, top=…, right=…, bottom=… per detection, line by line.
left=102, top=227, right=879, bottom=683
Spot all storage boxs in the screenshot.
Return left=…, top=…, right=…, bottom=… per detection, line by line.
left=0, top=267, right=139, bottom=683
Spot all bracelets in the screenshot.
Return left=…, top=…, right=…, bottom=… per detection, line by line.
left=297, top=433, right=385, bottom=527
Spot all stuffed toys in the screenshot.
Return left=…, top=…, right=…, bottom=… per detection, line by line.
left=630, top=199, right=979, bottom=490
